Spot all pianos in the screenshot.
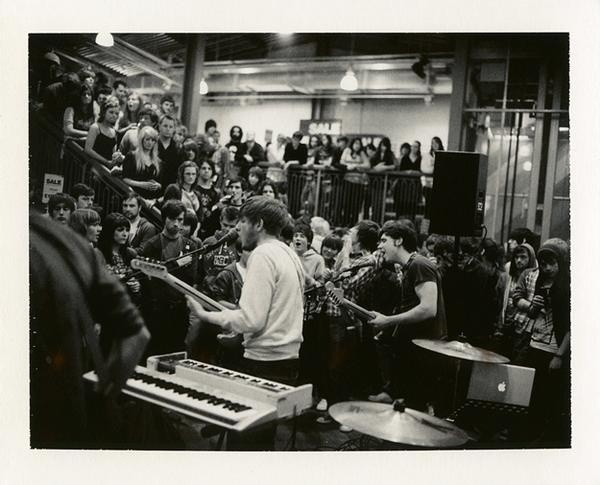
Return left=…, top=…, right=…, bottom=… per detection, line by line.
left=83, top=350, right=313, bottom=451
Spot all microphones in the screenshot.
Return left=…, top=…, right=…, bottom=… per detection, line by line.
left=341, top=256, right=376, bottom=273
left=202, top=228, right=239, bottom=253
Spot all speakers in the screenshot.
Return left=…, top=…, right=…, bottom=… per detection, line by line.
left=428, top=152, right=488, bottom=237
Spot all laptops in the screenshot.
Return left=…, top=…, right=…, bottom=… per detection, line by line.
left=466, top=362, right=536, bottom=406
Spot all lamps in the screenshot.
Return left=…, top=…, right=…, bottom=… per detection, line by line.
left=339, top=33, right=359, bottom=89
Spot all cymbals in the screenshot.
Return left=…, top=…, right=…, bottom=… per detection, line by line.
left=412, top=339, right=511, bottom=362
left=329, top=401, right=468, bottom=447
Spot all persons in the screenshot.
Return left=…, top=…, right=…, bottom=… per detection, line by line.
left=30, top=64, right=570, bottom=448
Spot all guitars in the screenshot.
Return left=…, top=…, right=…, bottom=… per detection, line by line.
left=130, top=256, right=240, bottom=312
left=326, top=288, right=405, bottom=343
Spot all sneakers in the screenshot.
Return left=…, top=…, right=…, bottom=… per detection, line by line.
left=368, top=393, right=393, bottom=402
left=317, top=416, right=333, bottom=424
left=317, top=399, right=327, bottom=410
